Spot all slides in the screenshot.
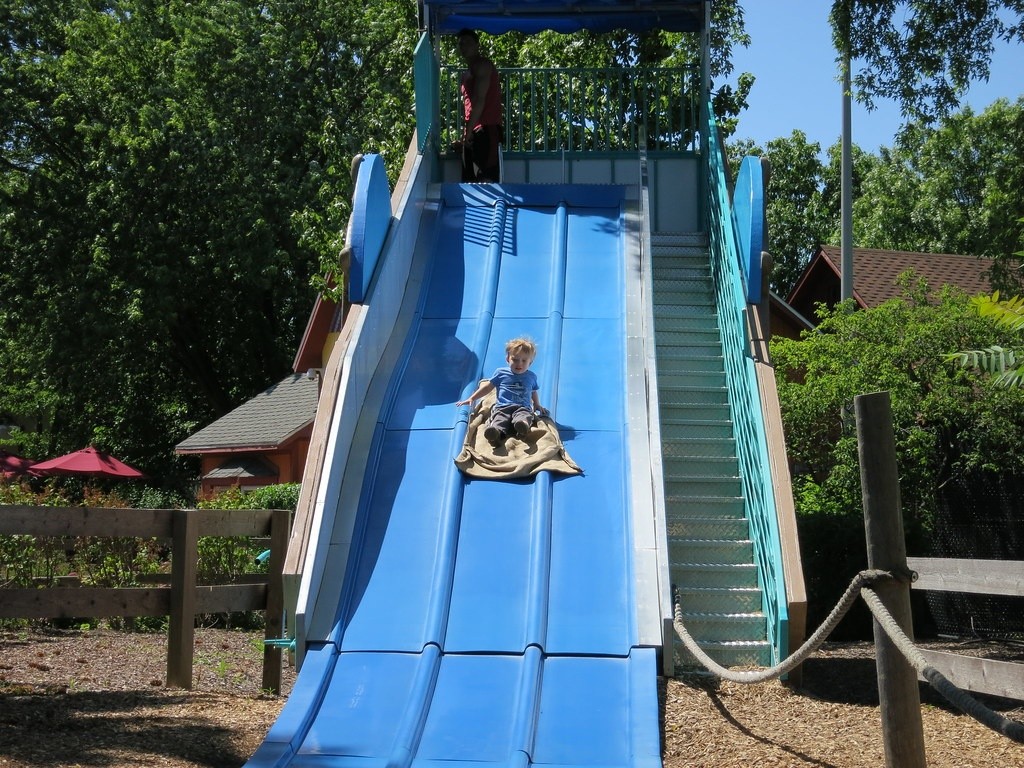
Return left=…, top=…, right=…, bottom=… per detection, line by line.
left=245, top=181, right=665, bottom=767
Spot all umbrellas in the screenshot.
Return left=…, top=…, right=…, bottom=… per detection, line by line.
left=0, top=447, right=144, bottom=479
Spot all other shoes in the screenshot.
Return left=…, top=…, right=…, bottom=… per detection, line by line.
left=484, top=427, right=502, bottom=448
left=514, top=420, right=529, bottom=438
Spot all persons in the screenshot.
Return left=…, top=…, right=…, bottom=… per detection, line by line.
left=455, top=28, right=501, bottom=184
left=455, top=337, right=549, bottom=448
